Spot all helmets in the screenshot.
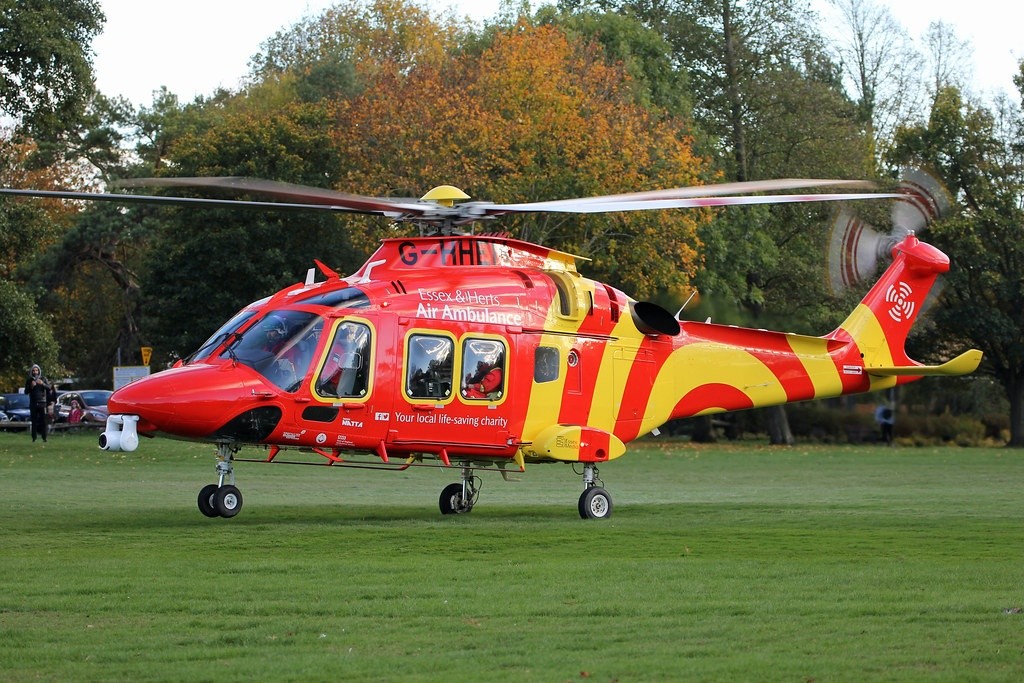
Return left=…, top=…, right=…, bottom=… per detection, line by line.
left=311, top=322, right=322, bottom=332
left=263, top=314, right=290, bottom=337
left=71, top=400, right=78, bottom=404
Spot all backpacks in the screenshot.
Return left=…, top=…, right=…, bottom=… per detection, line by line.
left=28, top=377, right=47, bottom=400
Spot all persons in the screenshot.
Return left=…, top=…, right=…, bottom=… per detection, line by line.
left=69, top=400, right=81, bottom=433
left=300, top=324, right=341, bottom=395
left=264, top=315, right=296, bottom=373
left=876, top=405, right=895, bottom=441
left=24, top=364, right=52, bottom=442
left=46, top=379, right=57, bottom=424
left=462, top=355, right=503, bottom=397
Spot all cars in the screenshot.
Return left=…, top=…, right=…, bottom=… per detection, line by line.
left=49, top=390, right=70, bottom=403
left=0, top=393, right=32, bottom=433
left=52, top=389, right=114, bottom=426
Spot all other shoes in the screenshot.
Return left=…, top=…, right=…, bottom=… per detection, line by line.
left=32, top=439, right=36, bottom=442
left=43, top=439, right=47, bottom=442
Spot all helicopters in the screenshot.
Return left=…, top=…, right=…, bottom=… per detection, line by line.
left=0, top=148, right=984, bottom=520
